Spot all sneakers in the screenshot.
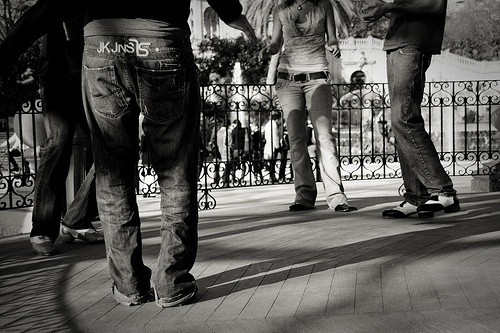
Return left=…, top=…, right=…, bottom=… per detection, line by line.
left=60, top=222, right=104, bottom=242
left=30, top=235, right=56, bottom=256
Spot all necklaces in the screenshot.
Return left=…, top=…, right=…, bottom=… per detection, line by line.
left=294, top=0, right=308, bottom=10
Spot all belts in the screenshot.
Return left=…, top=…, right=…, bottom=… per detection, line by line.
left=277, top=71, right=330, bottom=83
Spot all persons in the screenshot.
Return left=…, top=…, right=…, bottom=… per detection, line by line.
left=0, top=132, right=31, bottom=174
left=261, top=0, right=350, bottom=212
left=202, top=114, right=290, bottom=184
left=0, top=0, right=258, bottom=309
left=30, top=26, right=104, bottom=256
left=359, top=0, right=461, bottom=219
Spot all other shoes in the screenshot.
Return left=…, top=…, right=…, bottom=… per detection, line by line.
left=336, top=204, right=352, bottom=212
left=289, top=202, right=309, bottom=211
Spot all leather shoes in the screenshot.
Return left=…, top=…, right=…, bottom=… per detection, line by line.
left=417, top=194, right=460, bottom=214
left=381, top=200, right=435, bottom=219
